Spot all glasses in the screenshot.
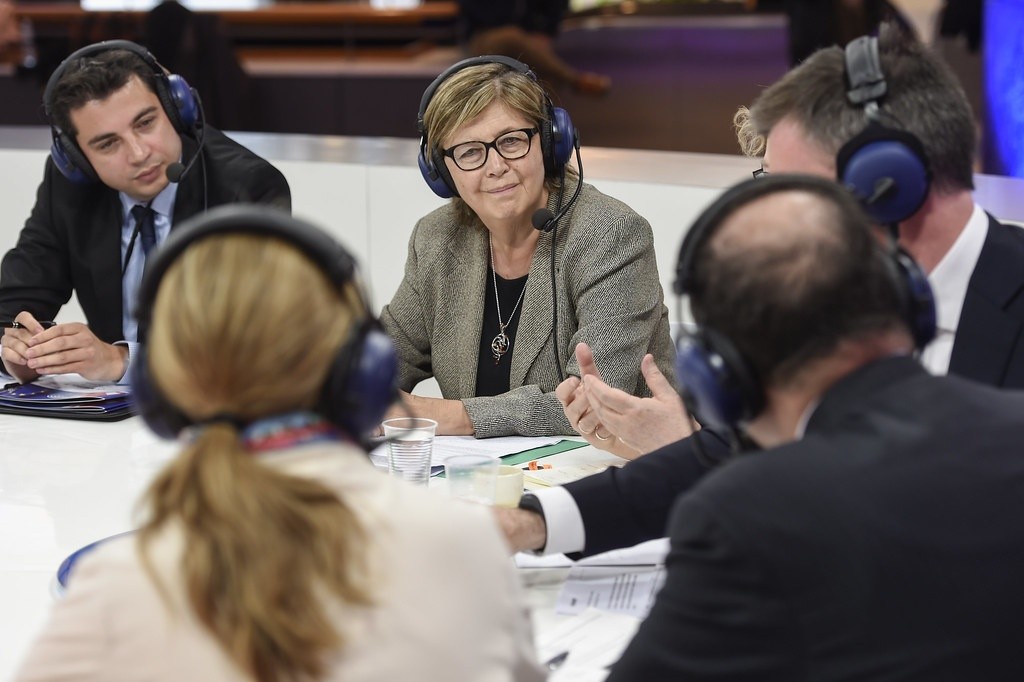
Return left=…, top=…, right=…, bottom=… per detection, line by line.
left=441, top=125, right=539, bottom=172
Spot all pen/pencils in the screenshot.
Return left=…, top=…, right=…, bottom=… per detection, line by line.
left=0, top=320, right=57, bottom=328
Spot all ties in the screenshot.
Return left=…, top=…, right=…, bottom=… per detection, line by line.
left=132, top=204, right=156, bottom=254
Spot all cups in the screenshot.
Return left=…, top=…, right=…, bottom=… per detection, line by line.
left=382, top=418, right=437, bottom=486
left=475, top=466, right=523, bottom=510
left=442, top=455, right=500, bottom=504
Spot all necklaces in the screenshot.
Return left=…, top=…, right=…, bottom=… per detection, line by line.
left=489, top=230, right=529, bottom=367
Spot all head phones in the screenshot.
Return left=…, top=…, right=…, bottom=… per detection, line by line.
left=44, top=40, right=203, bottom=189
left=416, top=55, right=584, bottom=200
left=670, top=172, right=939, bottom=429
left=834, top=34, right=934, bottom=228
left=128, top=203, right=405, bottom=436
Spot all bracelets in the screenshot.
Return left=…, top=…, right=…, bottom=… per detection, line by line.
left=519, top=494, right=543, bottom=514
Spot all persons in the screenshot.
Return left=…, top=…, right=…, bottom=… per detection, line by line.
left=790, top=0, right=996, bottom=167
left=601, top=171, right=1024, bottom=682
left=460, top=0, right=610, bottom=101
left=493, top=22, right=1024, bottom=563
left=8, top=201, right=552, bottom=682
left=377, top=53, right=680, bottom=439
left=0, top=40, right=295, bottom=388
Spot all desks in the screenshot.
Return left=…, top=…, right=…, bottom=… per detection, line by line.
left=1, top=411, right=673, bottom=681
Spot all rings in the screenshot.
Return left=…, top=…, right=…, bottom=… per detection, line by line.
left=595, top=426, right=608, bottom=441
left=577, top=421, right=590, bottom=435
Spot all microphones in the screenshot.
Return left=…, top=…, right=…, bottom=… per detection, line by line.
left=165, top=134, right=202, bottom=184
left=361, top=398, right=417, bottom=453
left=530, top=150, right=584, bottom=232
